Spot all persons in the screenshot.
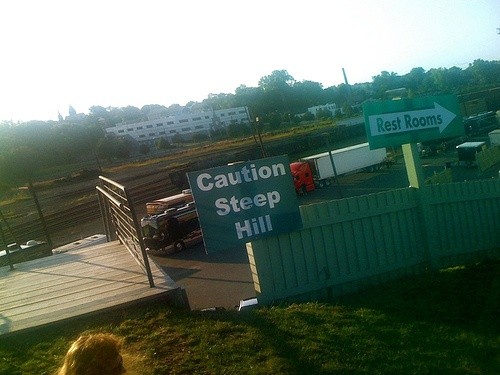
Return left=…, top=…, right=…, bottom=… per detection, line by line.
left=55, top=332, right=129, bottom=374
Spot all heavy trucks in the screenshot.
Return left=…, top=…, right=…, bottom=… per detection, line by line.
left=455, top=129, right=499, bottom=176
left=417, top=110, right=499, bottom=158
left=289, top=139, right=390, bottom=195
left=139, top=188, right=203, bottom=259
